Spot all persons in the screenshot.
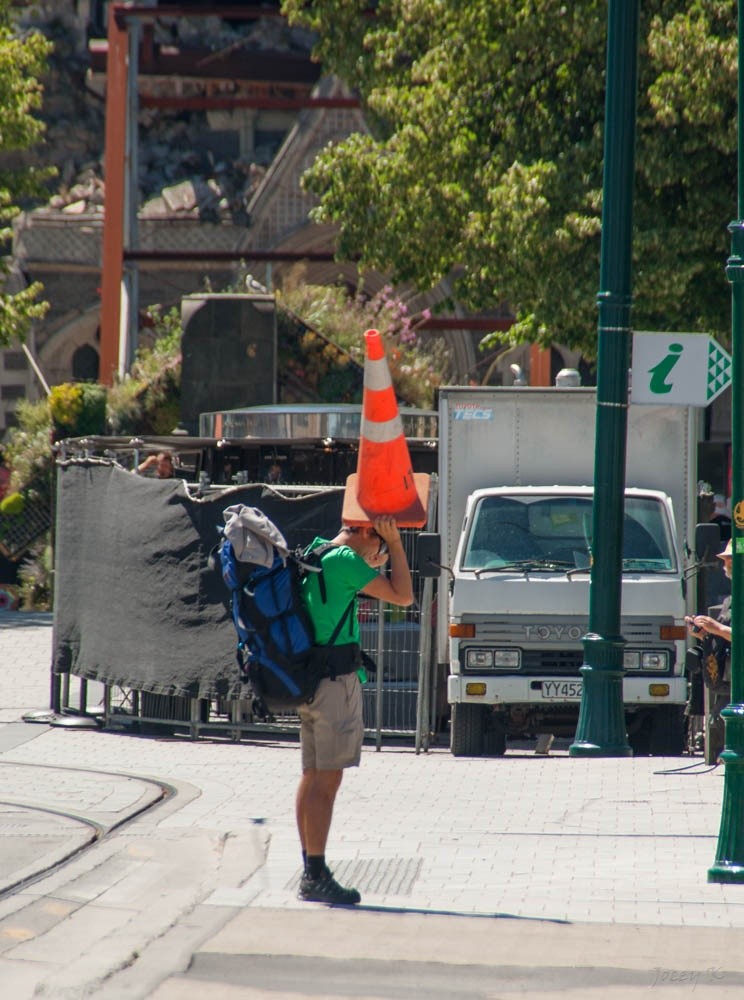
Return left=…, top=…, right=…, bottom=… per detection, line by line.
left=684, top=538, right=744, bottom=766
left=698, top=480, right=731, bottom=541
left=129, top=452, right=175, bottom=480
left=288, top=514, right=414, bottom=903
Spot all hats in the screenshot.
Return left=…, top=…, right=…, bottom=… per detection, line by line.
left=716, top=538, right=732, bottom=559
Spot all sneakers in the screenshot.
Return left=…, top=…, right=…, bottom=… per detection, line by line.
left=299, top=865, right=361, bottom=904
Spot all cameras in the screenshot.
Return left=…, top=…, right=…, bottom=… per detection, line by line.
left=690, top=620, right=703, bottom=634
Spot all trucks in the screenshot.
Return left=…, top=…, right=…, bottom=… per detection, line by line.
left=414, top=384, right=722, bottom=759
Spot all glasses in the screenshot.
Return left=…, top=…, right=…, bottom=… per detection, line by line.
left=379, top=540, right=388, bottom=554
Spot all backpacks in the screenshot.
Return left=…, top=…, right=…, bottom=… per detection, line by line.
left=208, top=539, right=355, bottom=707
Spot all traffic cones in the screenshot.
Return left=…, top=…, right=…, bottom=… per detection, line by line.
left=341, top=328, right=431, bottom=528
